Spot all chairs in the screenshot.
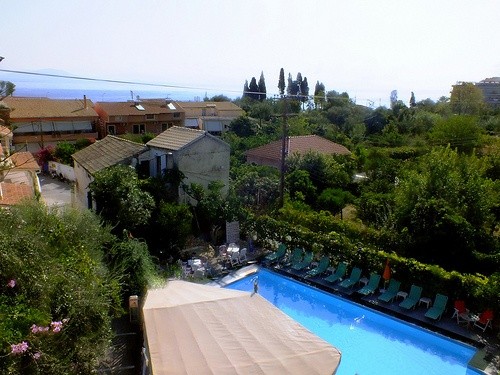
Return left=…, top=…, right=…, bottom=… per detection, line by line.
left=399, top=285, right=422, bottom=309
left=376, top=278, right=401, bottom=303
left=291, top=252, right=313, bottom=272
left=187, top=242, right=247, bottom=276
left=424, top=294, right=449, bottom=320
left=339, top=267, right=362, bottom=290
left=265, top=244, right=286, bottom=263
left=325, top=261, right=349, bottom=284
left=280, top=248, right=302, bottom=268
left=452, top=301, right=470, bottom=328
left=356, top=273, right=381, bottom=296
left=306, top=257, right=329, bottom=277
left=473, top=309, right=493, bottom=332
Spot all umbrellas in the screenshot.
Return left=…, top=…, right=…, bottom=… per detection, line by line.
left=247, top=237, right=253, bottom=255
left=383, top=256, right=390, bottom=291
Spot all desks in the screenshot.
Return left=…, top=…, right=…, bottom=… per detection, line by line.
left=327, top=267, right=336, bottom=275
left=419, top=298, right=432, bottom=309
left=359, top=278, right=368, bottom=286
left=396, top=291, right=408, bottom=301
